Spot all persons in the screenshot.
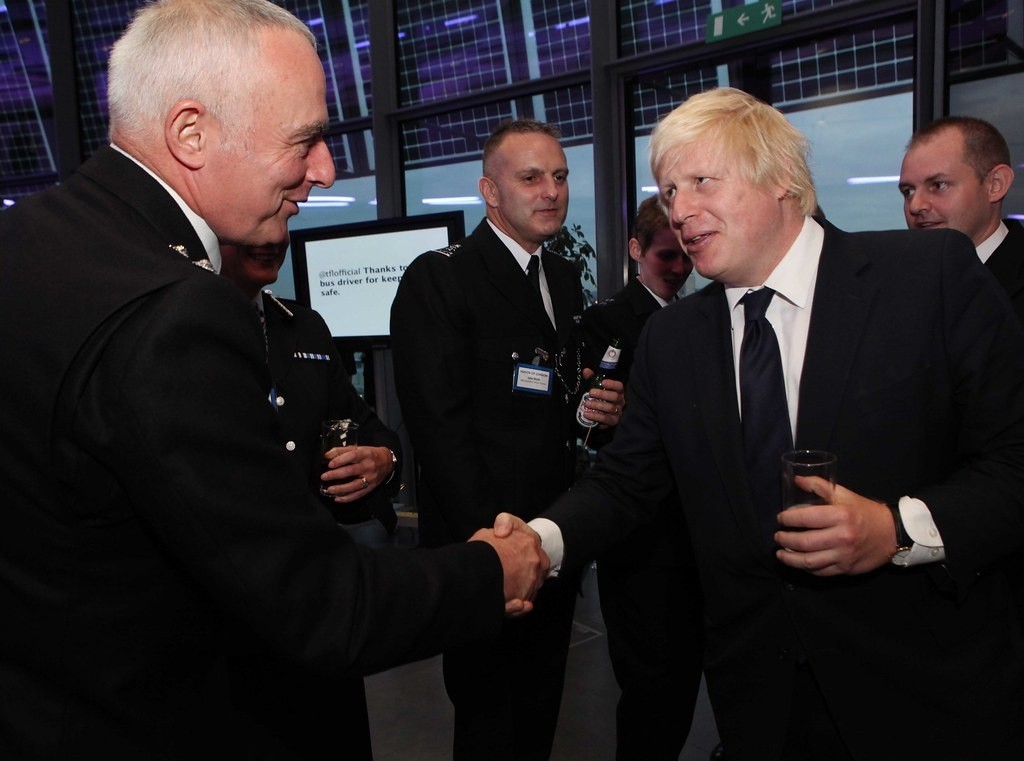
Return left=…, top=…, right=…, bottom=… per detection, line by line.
left=0, top=1, right=550, bottom=761
left=489, top=86, right=1023, bottom=757
left=577, top=188, right=709, bottom=756
left=392, top=116, right=628, bottom=760
left=888, top=112, right=1022, bottom=276
left=202, top=230, right=407, bottom=566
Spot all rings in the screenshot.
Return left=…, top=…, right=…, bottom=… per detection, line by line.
left=359, top=475, right=369, bottom=488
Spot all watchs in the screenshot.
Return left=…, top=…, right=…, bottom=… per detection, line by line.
left=384, top=448, right=401, bottom=489
left=884, top=495, right=913, bottom=559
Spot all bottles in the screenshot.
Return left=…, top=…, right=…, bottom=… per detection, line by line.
left=565, top=333, right=628, bottom=441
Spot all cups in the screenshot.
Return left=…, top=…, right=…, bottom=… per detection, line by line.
left=780, top=448, right=839, bottom=535
left=319, top=418, right=361, bottom=498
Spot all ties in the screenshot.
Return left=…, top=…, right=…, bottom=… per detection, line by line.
left=527, top=254, right=556, bottom=342
left=738, top=285, right=798, bottom=558
